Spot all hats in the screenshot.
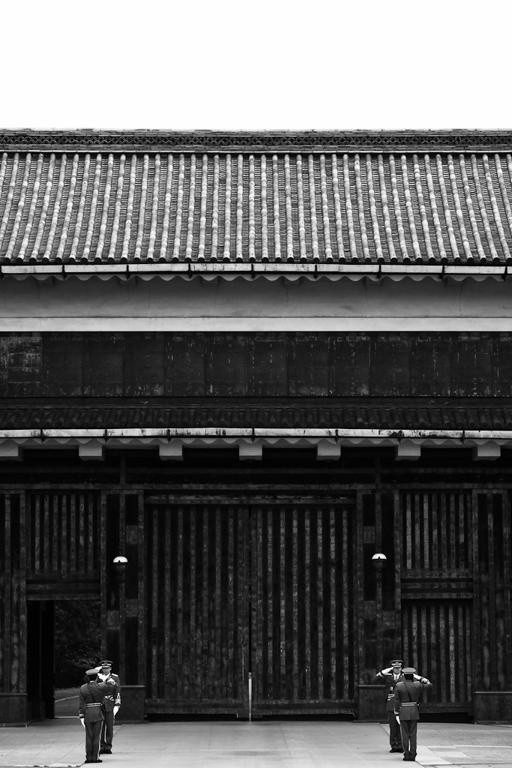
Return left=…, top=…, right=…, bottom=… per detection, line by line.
left=391, top=660, right=416, bottom=674
left=85, top=660, right=113, bottom=675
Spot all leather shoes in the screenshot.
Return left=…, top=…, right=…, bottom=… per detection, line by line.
left=390, top=748, right=415, bottom=761
left=85, top=750, right=112, bottom=763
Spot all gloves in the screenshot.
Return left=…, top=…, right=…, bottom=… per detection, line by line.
left=113, top=706, right=120, bottom=716
left=98, top=673, right=107, bottom=681
left=412, top=673, right=421, bottom=680
left=382, top=667, right=393, bottom=676
left=80, top=718, right=85, bottom=728
left=396, top=715, right=400, bottom=725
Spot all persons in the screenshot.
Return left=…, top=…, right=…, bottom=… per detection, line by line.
left=78, top=660, right=122, bottom=763
left=376, top=659, right=433, bottom=761
